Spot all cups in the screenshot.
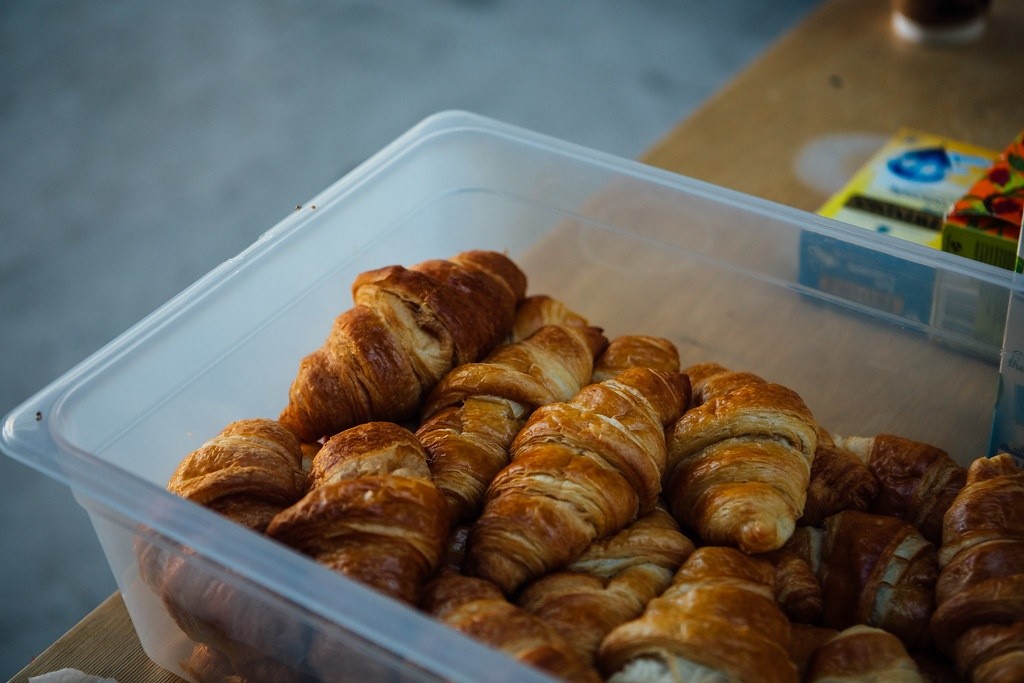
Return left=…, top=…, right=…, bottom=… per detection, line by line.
left=890, top=0, right=993, bottom=48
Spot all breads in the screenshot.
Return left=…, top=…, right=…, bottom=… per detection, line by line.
left=130, top=250, right=1024, bottom=683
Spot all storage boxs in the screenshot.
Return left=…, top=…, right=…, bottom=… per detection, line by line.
left=792, top=121, right=1006, bottom=341
left=2, top=110, right=1023, bottom=683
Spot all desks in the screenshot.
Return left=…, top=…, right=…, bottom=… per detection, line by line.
left=0, top=0, right=1024, bottom=683
